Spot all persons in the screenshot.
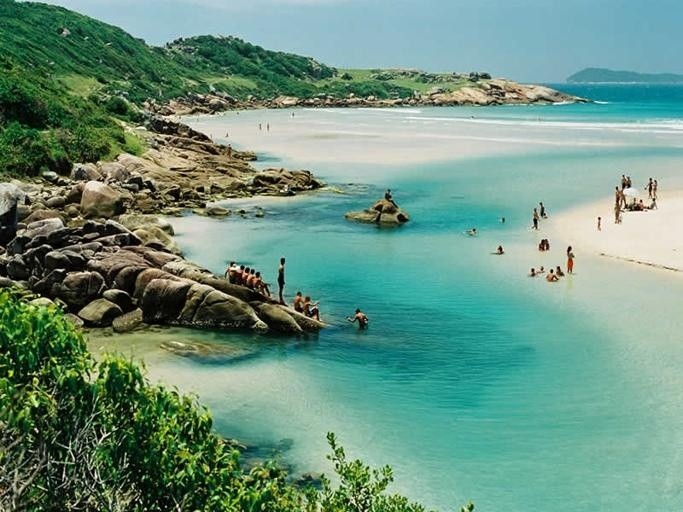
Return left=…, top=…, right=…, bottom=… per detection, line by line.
left=465, top=228, right=477, bottom=236
left=543, top=213, right=548, bottom=218
left=277, top=258, right=288, bottom=307
left=566, top=246, right=574, bottom=273
left=501, top=217, right=504, bottom=225
left=538, top=238, right=545, bottom=252
left=496, top=245, right=503, bottom=254
left=531, top=208, right=540, bottom=230
left=347, top=308, right=369, bottom=330
left=292, top=291, right=312, bottom=313
left=528, top=264, right=563, bottom=282
left=538, top=202, right=544, bottom=217
left=613, top=174, right=657, bottom=224
left=597, top=217, right=601, bottom=229
left=223, top=261, right=270, bottom=298
left=303, top=295, right=322, bottom=322
left=543, top=239, right=549, bottom=251
left=384, top=189, right=399, bottom=210
